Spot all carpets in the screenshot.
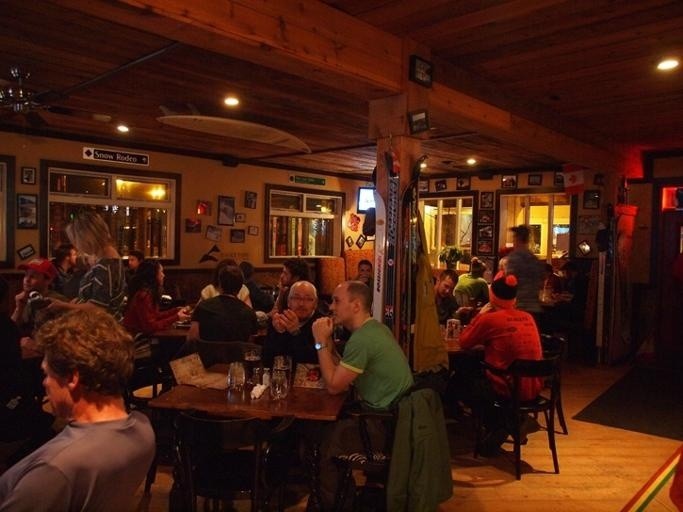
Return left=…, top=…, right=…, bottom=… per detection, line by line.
left=571, top=357, right=683, bottom=441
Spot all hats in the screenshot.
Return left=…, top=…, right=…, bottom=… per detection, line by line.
left=559, top=260, right=578, bottom=269
left=19, top=258, right=57, bottom=277
left=510, top=225, right=531, bottom=236
left=489, top=275, right=518, bottom=306
left=471, top=257, right=486, bottom=273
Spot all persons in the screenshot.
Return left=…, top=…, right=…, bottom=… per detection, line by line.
left=355, top=260, right=374, bottom=290
left=433, top=225, right=588, bottom=325
left=312, top=281, right=415, bottom=512
left=447, top=273, right=545, bottom=457
left=0, top=308, right=156, bottom=512
left=0, top=211, right=191, bottom=443
left=185, top=257, right=324, bottom=364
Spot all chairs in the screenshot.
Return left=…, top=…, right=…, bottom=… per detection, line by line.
left=332, top=389, right=453, bottom=512
left=120, top=352, right=176, bottom=413
left=540, top=333, right=567, bottom=434
left=194, top=336, right=256, bottom=369
left=175, top=410, right=286, bottom=512
left=479, top=357, right=562, bottom=479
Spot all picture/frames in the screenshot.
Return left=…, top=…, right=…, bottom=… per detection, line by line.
left=409, top=54, right=433, bottom=88
left=216, top=191, right=259, bottom=243
left=583, top=190, right=600, bottom=209
left=346, top=235, right=366, bottom=249
left=477, top=191, right=495, bottom=254
left=17, top=166, right=38, bottom=260
left=435, top=175, right=471, bottom=192
left=501, top=174, right=542, bottom=191
left=408, top=108, right=429, bottom=134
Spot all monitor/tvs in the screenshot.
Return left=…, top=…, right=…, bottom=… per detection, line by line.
left=356, top=185, right=380, bottom=212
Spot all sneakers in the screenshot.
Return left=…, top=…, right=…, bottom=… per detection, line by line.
left=520, top=415, right=539, bottom=444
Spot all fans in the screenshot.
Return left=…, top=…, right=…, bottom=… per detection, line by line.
left=0, top=66, right=112, bottom=127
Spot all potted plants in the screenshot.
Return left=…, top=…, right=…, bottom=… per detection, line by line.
left=438, top=244, right=463, bottom=270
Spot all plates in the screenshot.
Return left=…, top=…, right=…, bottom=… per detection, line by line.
left=177, top=323, right=192, bottom=328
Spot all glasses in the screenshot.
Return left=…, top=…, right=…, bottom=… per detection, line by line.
left=291, top=297, right=314, bottom=303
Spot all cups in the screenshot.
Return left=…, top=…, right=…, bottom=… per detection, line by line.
left=244, top=350, right=261, bottom=378
left=271, top=353, right=292, bottom=388
left=227, top=361, right=245, bottom=389
left=269, top=370, right=288, bottom=401
left=445, top=318, right=461, bottom=339
left=227, top=389, right=246, bottom=405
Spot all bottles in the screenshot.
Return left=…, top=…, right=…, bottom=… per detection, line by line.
left=252, top=367, right=261, bottom=386
left=262, top=367, right=271, bottom=386
